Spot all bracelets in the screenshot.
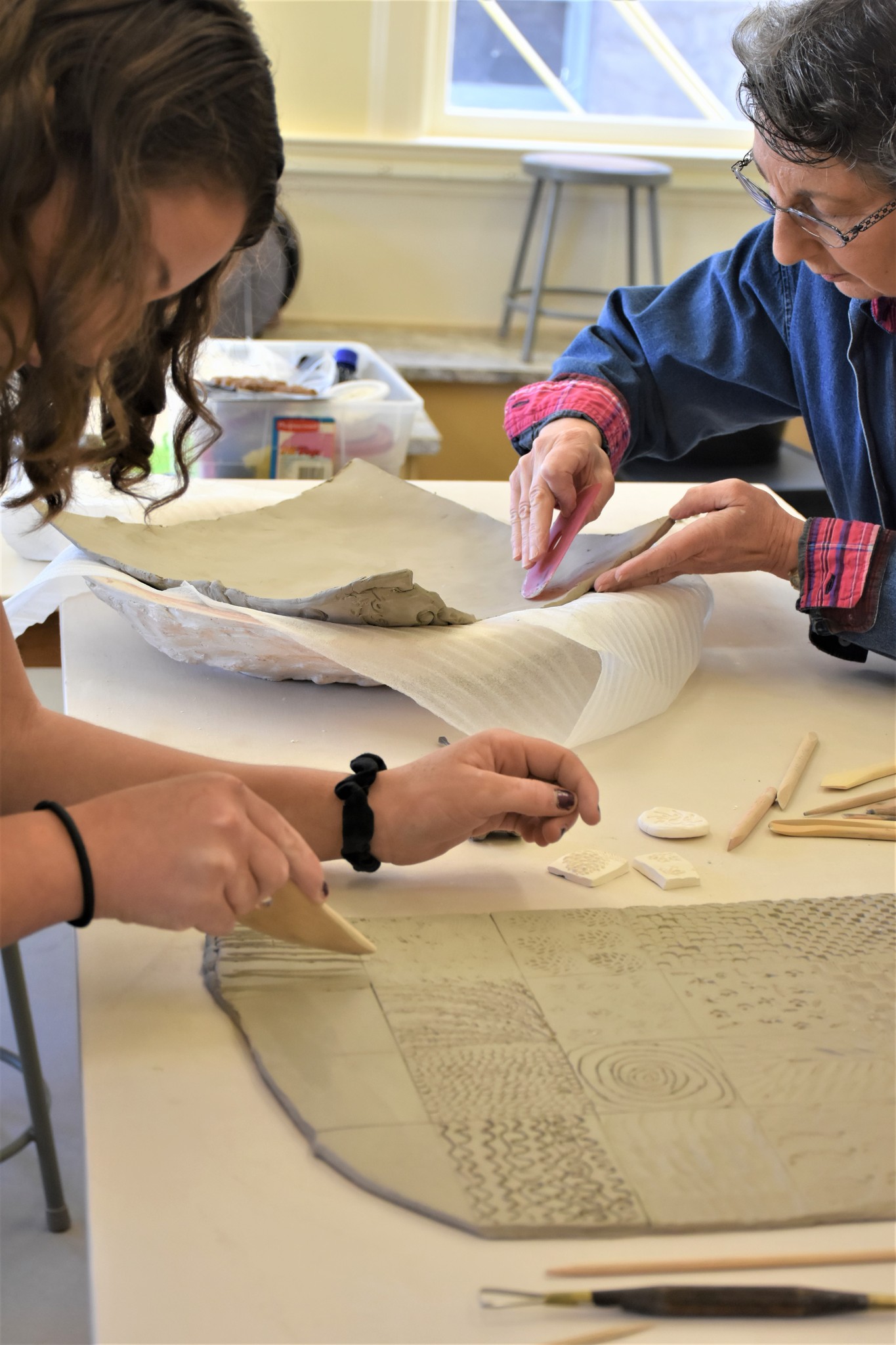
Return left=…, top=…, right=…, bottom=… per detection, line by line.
left=334, top=753, right=387, bottom=873
left=35, top=800, right=94, bottom=927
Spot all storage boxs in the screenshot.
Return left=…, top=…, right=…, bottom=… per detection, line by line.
left=172, top=331, right=427, bottom=487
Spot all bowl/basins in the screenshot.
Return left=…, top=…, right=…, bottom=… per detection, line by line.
left=85, top=576, right=388, bottom=689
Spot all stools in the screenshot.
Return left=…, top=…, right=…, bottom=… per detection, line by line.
left=499, top=154, right=674, bottom=364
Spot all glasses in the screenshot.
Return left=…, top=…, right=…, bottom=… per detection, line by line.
left=731, top=148, right=896, bottom=248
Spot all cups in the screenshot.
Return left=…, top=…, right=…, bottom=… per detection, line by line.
left=324, top=380, right=390, bottom=441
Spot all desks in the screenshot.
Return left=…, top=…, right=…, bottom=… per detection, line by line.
left=54, top=476, right=896, bottom=1345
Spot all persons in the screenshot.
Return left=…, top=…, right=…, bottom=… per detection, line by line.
left=505, top=0, right=896, bottom=665
left=0, top=0, right=600, bottom=952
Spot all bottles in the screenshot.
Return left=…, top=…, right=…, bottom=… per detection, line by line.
left=334, top=349, right=359, bottom=381
